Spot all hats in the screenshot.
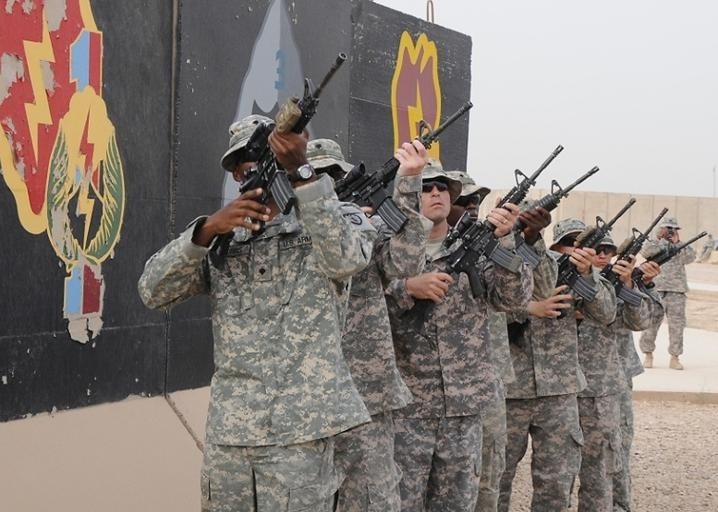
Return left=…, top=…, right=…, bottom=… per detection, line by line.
left=422, top=159, right=490, bottom=205
left=659, top=215, right=681, bottom=229
left=220, top=115, right=276, bottom=172
left=601, top=233, right=617, bottom=251
left=549, top=218, right=586, bottom=250
left=305, top=139, right=355, bottom=172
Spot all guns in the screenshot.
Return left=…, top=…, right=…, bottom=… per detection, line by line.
left=554, top=198, right=637, bottom=319
left=334, top=100, right=473, bottom=232
left=209, top=52, right=347, bottom=271
left=398, top=146, right=565, bottom=339
left=627, top=231, right=706, bottom=293
left=599, top=207, right=668, bottom=306
left=511, top=166, right=600, bottom=271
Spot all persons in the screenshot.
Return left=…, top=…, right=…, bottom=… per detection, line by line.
left=383, top=158, right=535, bottom=512
left=639, top=215, right=697, bottom=370
left=137, top=114, right=379, bottom=512
left=304, top=138, right=428, bottom=512
left=696, top=234, right=714, bottom=263
left=445, top=169, right=661, bottom=511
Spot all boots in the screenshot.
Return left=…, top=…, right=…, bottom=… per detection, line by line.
left=643, top=353, right=653, bottom=367
left=670, top=356, right=682, bottom=369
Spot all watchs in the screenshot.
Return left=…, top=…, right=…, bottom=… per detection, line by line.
left=287, top=163, right=316, bottom=183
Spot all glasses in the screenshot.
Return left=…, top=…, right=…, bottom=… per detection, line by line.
left=596, top=246, right=610, bottom=254
left=562, top=236, right=576, bottom=246
left=240, top=147, right=260, bottom=163
